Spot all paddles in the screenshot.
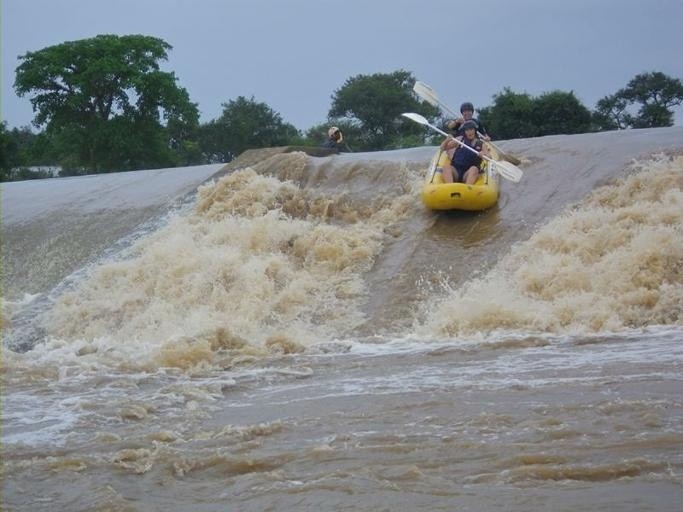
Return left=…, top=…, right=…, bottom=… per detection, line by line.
left=402, top=113, right=523, bottom=184
left=412, top=81, right=520, bottom=167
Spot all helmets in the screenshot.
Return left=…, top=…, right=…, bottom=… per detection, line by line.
left=460, top=102, right=475, bottom=115
left=461, top=121, right=479, bottom=131
left=328, top=127, right=344, bottom=143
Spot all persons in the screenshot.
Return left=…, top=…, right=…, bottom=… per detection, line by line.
left=447, top=103, right=491, bottom=140
left=329, top=127, right=350, bottom=153
left=441, top=120, right=487, bottom=184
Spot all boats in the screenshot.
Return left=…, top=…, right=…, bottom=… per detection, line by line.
left=419, top=138, right=500, bottom=214
